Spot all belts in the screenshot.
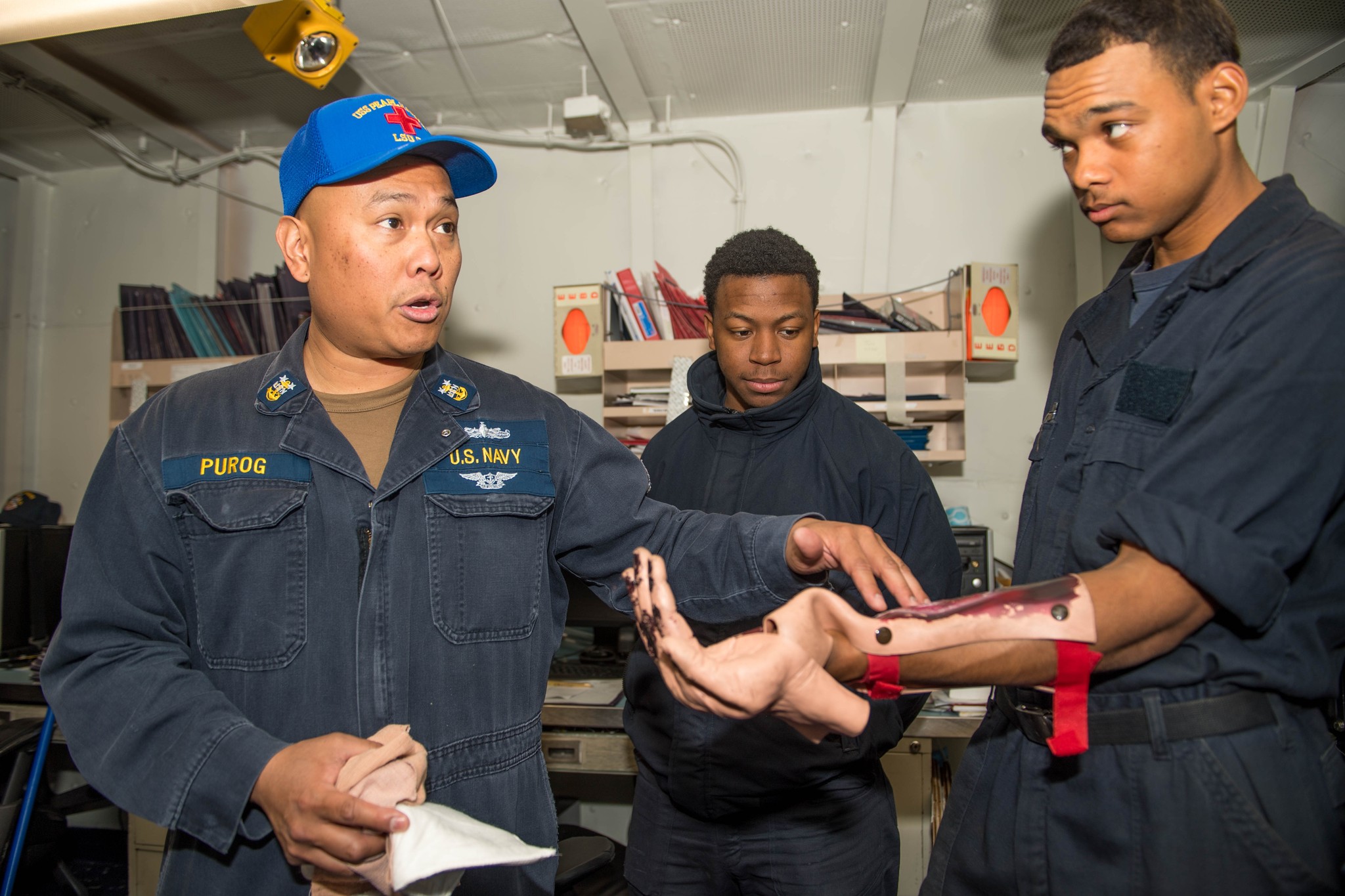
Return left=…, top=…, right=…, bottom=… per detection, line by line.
left=995, top=684, right=1304, bottom=748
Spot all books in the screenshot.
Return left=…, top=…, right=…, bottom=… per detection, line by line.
left=614, top=385, right=671, bottom=407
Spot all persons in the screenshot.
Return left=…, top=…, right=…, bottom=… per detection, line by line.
left=620, top=544, right=1099, bottom=748
left=622, top=228, right=963, bottom=896
left=825, top=0, right=1345, bottom=896
left=36, top=94, right=932, bottom=896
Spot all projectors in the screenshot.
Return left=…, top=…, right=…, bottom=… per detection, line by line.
left=563, top=94, right=613, bottom=138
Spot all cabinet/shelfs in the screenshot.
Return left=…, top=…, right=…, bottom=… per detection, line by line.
left=104, top=305, right=264, bottom=441
left=123, top=680, right=993, bottom=896
left=598, top=263, right=969, bottom=473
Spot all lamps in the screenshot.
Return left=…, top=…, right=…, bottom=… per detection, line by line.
left=240, top=0, right=361, bottom=91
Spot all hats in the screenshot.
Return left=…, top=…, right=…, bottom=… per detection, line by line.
left=280, top=94, right=497, bottom=217
